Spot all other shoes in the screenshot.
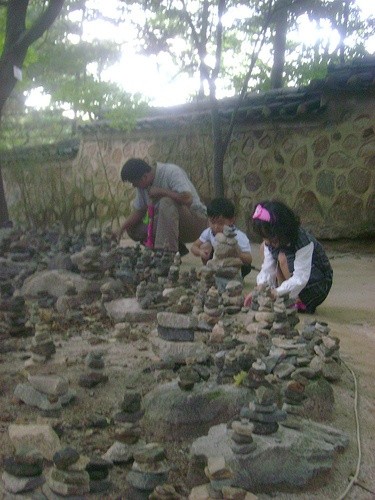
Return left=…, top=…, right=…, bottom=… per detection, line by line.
left=295, top=300, right=316, bottom=314
left=177, top=243, right=189, bottom=256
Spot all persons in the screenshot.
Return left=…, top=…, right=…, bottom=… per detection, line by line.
left=245, top=200, right=334, bottom=313
left=191, top=198, right=252, bottom=279
left=110, top=159, right=208, bottom=257
